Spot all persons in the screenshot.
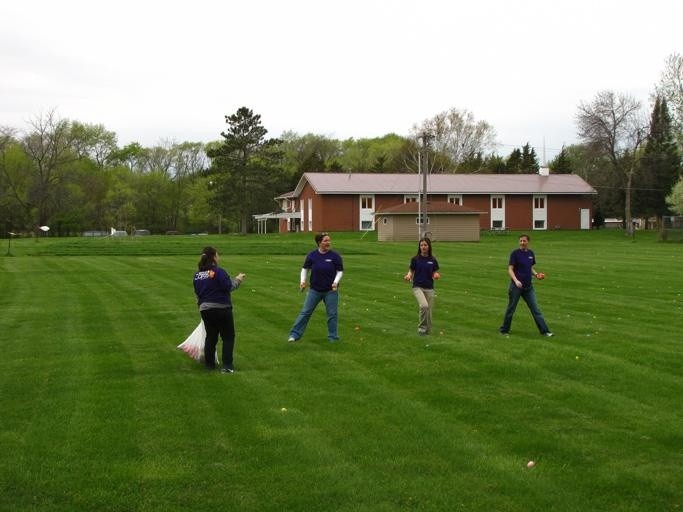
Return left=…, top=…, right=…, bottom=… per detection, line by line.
left=403, top=237, right=440, bottom=336
left=287, top=232, right=343, bottom=344
left=193, top=246, right=245, bottom=375
left=500, top=236, right=554, bottom=338
